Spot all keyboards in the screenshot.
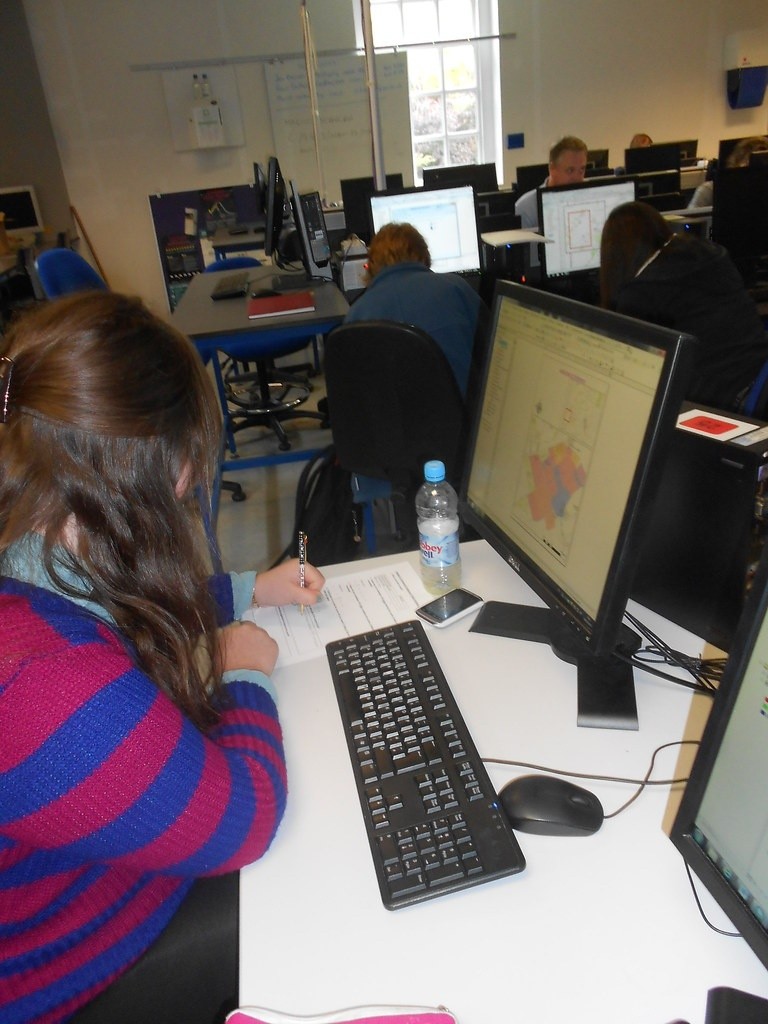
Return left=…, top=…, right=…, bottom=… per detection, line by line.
left=228, top=225, right=248, bottom=235
left=210, top=270, right=250, bottom=301
left=325, top=619, right=527, bottom=911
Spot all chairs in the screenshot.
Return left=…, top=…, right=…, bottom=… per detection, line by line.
left=34, top=247, right=247, bottom=504
left=206, top=256, right=329, bottom=452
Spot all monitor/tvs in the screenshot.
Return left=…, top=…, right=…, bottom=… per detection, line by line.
left=0, top=185, right=45, bottom=237
left=666, top=553, right=768, bottom=1024
left=339, top=138, right=768, bottom=1024
left=252, top=156, right=333, bottom=282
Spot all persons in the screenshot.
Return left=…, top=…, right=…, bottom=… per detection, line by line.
left=514, top=135, right=588, bottom=229
left=685, top=136, right=768, bottom=208
left=599, top=201, right=768, bottom=419
left=1, top=289, right=325, bottom=1024
left=343, top=223, right=482, bottom=397
left=630, top=133, right=654, bottom=149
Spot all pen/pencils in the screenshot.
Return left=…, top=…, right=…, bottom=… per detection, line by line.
left=297, top=531, right=307, bottom=615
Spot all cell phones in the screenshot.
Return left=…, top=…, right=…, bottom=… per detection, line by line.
left=415, top=587, right=484, bottom=628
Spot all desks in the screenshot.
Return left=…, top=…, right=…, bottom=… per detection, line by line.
left=170, top=266, right=352, bottom=577
left=241, top=538, right=768, bottom=1024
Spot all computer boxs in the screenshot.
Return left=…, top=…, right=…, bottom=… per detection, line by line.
left=626, top=400, right=768, bottom=654
left=480, top=240, right=532, bottom=307
left=289, top=192, right=333, bottom=270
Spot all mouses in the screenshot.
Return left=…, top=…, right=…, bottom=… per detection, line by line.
left=498, top=774, right=604, bottom=837
left=251, top=288, right=281, bottom=298
